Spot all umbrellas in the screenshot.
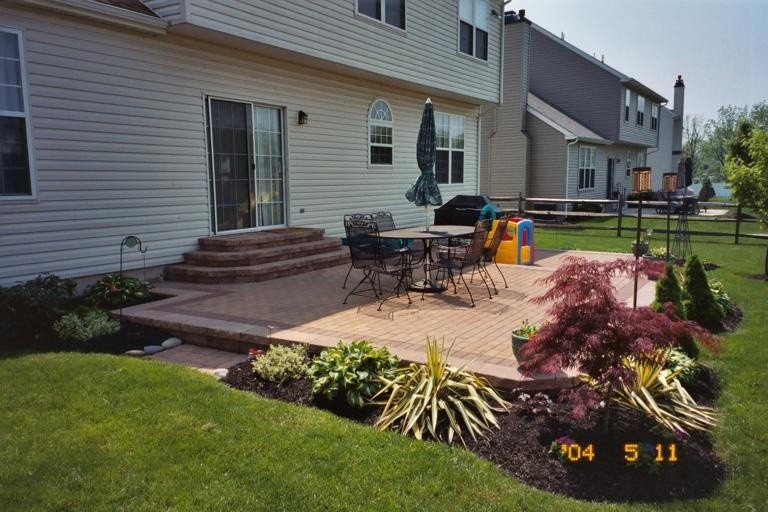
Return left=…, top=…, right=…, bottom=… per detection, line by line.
left=404, top=97, right=444, bottom=284
left=676, top=157, right=685, bottom=196
left=685, top=156, right=692, bottom=196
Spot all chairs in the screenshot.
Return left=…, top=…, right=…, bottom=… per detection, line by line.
left=342, top=210, right=508, bottom=310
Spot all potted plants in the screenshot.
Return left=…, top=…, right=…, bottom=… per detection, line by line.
left=511, top=319, right=538, bottom=367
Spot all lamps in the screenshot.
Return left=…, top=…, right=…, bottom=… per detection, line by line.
left=491, top=9, right=502, bottom=18
left=299, top=110, right=307, bottom=124
